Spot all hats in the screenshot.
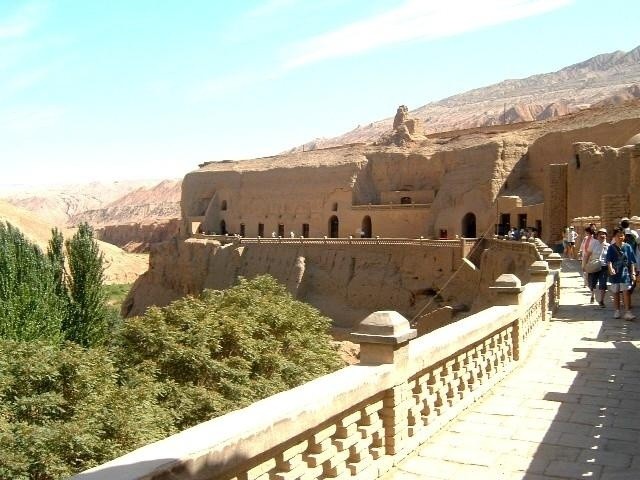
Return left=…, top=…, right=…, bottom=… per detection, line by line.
left=596, top=228, right=607, bottom=234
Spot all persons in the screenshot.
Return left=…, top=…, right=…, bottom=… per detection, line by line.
left=271, top=231, right=277, bottom=237
left=507, top=219, right=640, bottom=321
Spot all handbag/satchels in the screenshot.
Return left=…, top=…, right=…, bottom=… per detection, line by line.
left=585, top=259, right=601, bottom=273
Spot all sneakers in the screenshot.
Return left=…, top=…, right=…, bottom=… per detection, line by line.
left=599, top=301, right=605, bottom=307
left=624, top=312, right=636, bottom=320
left=615, top=310, right=621, bottom=318
left=591, top=298, right=594, bottom=304
